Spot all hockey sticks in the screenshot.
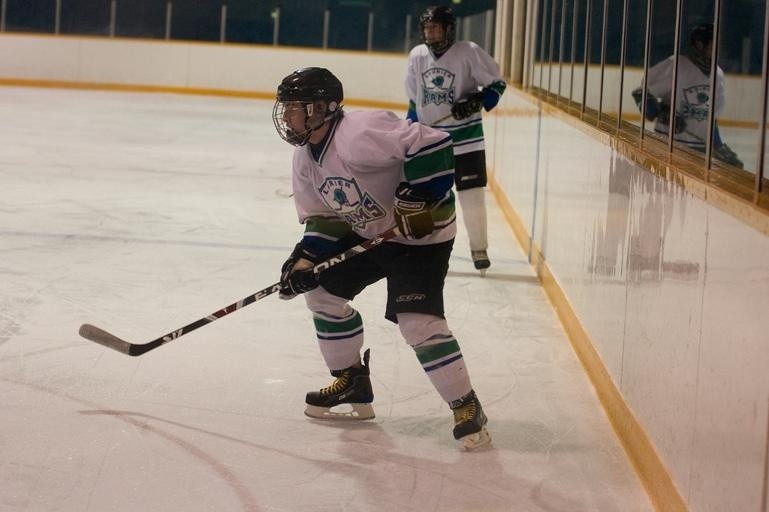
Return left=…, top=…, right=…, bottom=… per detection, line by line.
left=80, top=227, right=400, bottom=356
left=276, top=115, right=453, bottom=199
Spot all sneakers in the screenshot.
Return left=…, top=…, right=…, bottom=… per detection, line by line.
left=472, top=251, right=490, bottom=269
left=304, top=348, right=374, bottom=407
left=449, top=390, right=488, bottom=439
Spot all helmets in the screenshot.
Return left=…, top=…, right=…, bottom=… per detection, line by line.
left=273, top=68, right=343, bottom=146
left=420, top=1, right=454, bottom=52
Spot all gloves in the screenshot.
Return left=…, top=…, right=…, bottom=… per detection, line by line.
left=279, top=243, right=321, bottom=298
left=393, top=182, right=434, bottom=240
left=450, top=92, right=481, bottom=119
left=717, top=142, right=744, bottom=170
left=665, top=109, right=686, bottom=134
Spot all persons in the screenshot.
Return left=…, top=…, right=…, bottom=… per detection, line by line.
left=592, top=157, right=674, bottom=279
left=269, top=64, right=489, bottom=438
left=401, top=5, right=509, bottom=269
left=632, top=18, right=743, bottom=169
